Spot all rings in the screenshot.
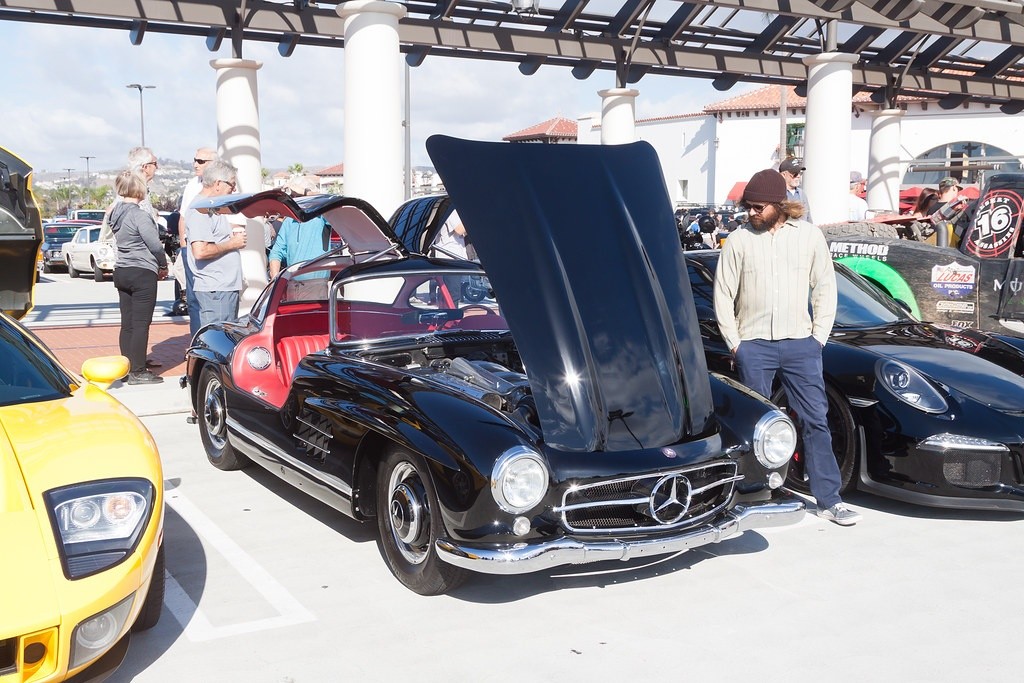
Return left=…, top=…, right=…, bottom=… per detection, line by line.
left=163, top=278, right=165, bottom=280
left=166, top=277, right=168, bottom=278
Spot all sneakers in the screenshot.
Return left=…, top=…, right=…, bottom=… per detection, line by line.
left=816, top=500, right=862, bottom=525
left=127, top=369, right=163, bottom=385
left=121, top=374, right=129, bottom=382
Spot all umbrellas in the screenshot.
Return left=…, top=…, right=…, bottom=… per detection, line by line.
left=899, top=187, right=980, bottom=216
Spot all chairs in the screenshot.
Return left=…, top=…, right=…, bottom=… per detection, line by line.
left=276, top=334, right=360, bottom=389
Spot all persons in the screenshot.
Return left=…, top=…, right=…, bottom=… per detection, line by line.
left=911, top=188, right=938, bottom=219
left=779, top=158, right=813, bottom=224
left=178, top=151, right=246, bottom=338
left=685, top=214, right=701, bottom=237
left=715, top=213, right=724, bottom=231
left=268, top=177, right=332, bottom=301
left=847, top=172, right=886, bottom=223
left=183, top=160, right=247, bottom=423
left=927, top=177, right=963, bottom=248
left=700, top=226, right=719, bottom=250
left=107, top=170, right=169, bottom=385
left=727, top=214, right=737, bottom=231
left=435, top=207, right=466, bottom=310
left=688, top=215, right=703, bottom=235
left=712, top=169, right=862, bottom=525
left=110, top=147, right=162, bottom=367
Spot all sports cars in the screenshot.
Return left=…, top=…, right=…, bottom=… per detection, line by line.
left=192, top=190, right=1024, bottom=515
left=0, top=147, right=165, bottom=683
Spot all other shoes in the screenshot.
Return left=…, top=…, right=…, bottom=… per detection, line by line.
left=162, top=311, right=176, bottom=316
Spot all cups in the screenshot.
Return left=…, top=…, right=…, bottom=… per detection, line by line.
left=232, top=227, right=245, bottom=236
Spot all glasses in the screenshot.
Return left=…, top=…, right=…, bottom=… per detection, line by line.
left=142, top=161, right=158, bottom=170
left=742, top=202, right=772, bottom=213
left=785, top=171, right=803, bottom=179
left=219, top=179, right=236, bottom=190
left=194, top=157, right=214, bottom=164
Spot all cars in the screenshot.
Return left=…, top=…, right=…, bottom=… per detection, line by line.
left=181, top=134, right=811, bottom=597
left=38, top=209, right=173, bottom=281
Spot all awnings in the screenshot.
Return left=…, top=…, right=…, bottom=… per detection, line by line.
left=726, top=182, right=748, bottom=201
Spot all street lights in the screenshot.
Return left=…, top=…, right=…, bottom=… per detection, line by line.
left=127, top=84, right=155, bottom=147
left=80, top=156, right=96, bottom=208
left=63, top=168, right=76, bottom=210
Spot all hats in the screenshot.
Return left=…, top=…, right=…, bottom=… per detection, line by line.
left=743, top=169, right=787, bottom=202
left=283, top=176, right=318, bottom=195
left=939, top=176, right=963, bottom=192
left=779, top=157, right=806, bottom=174
left=850, top=171, right=866, bottom=184
